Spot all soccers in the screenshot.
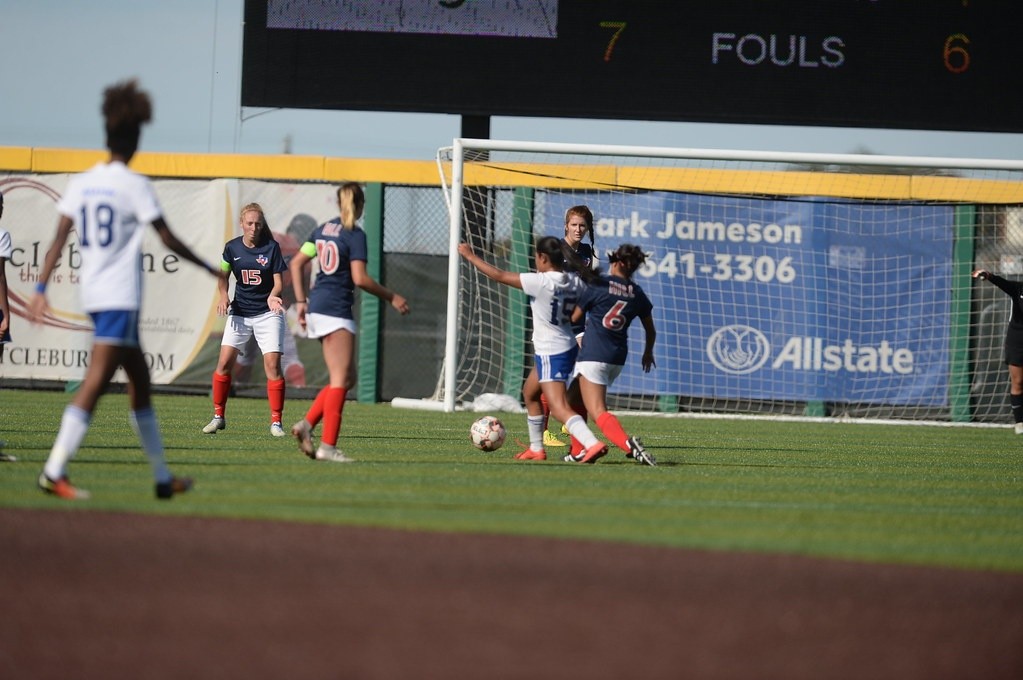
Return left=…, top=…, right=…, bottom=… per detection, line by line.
left=469, top=415, right=506, bottom=451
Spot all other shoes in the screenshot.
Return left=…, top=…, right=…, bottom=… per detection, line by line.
left=0, top=453, right=16, bottom=461
left=1015, top=423, right=1023, bottom=434
left=291, top=421, right=316, bottom=460
left=156, top=477, right=192, bottom=499
left=38, top=472, right=89, bottom=499
left=316, top=447, right=356, bottom=462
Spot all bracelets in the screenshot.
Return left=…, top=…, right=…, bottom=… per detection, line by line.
left=297, top=300, right=308, bottom=304
left=36, top=283, right=46, bottom=294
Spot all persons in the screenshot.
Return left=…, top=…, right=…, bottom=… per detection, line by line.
left=973, top=268, right=1023, bottom=434
left=291, top=183, right=410, bottom=461
left=457, top=236, right=608, bottom=464
left=234, top=213, right=319, bottom=388
left=565, top=243, right=656, bottom=467
left=29, top=83, right=227, bottom=498
left=558, top=205, right=601, bottom=286
left=0, top=192, right=16, bottom=460
left=202, top=202, right=287, bottom=437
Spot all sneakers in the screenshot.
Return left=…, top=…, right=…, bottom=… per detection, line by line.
left=581, top=441, right=609, bottom=463
left=626, top=436, right=658, bottom=467
left=514, top=448, right=547, bottom=460
left=203, top=418, right=225, bottom=434
left=270, top=423, right=286, bottom=437
left=560, top=449, right=586, bottom=463
